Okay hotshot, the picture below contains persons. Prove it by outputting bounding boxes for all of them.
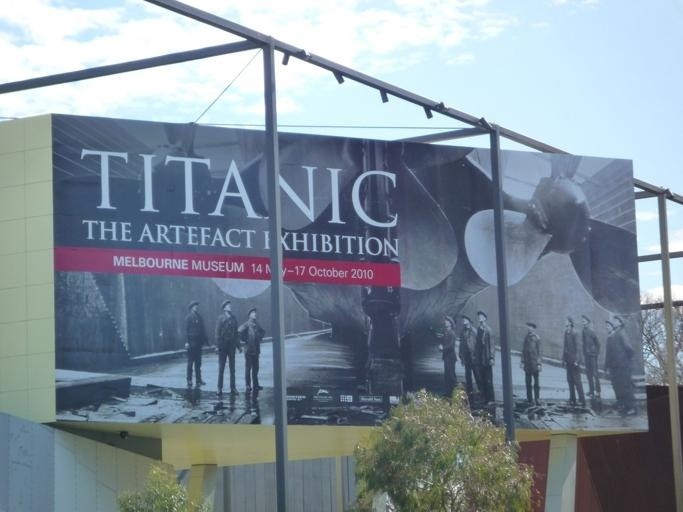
[237,307,266,391]
[183,300,210,387]
[561,316,587,407]
[519,322,542,407]
[603,320,625,412]
[459,313,483,407]
[612,314,637,415]
[474,310,496,407]
[214,299,243,396]
[581,314,602,397]
[429,314,458,397]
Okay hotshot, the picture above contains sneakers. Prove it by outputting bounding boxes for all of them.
[187,380,264,395]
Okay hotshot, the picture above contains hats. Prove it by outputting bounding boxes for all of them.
[526,320,537,327]
[566,315,575,324]
[188,300,199,309]
[220,299,230,308]
[445,311,486,327]
[605,315,626,328]
[247,307,257,317]
[582,314,591,322]
